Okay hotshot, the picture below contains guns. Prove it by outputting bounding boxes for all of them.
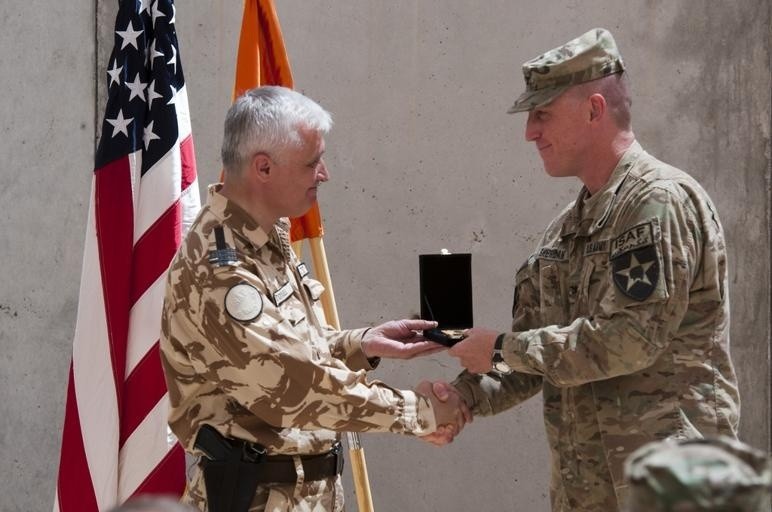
[194,423,267,512]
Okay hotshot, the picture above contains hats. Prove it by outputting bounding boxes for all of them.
[506,27,625,113]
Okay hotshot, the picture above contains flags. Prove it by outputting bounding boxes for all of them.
[47,0,200,510]
[212,2,322,274]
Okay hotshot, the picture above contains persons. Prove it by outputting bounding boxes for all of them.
[424,23,742,511]
[157,82,474,512]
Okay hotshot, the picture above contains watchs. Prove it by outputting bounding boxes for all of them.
[490,331,514,379]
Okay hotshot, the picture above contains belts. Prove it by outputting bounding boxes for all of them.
[196,442,345,482]
